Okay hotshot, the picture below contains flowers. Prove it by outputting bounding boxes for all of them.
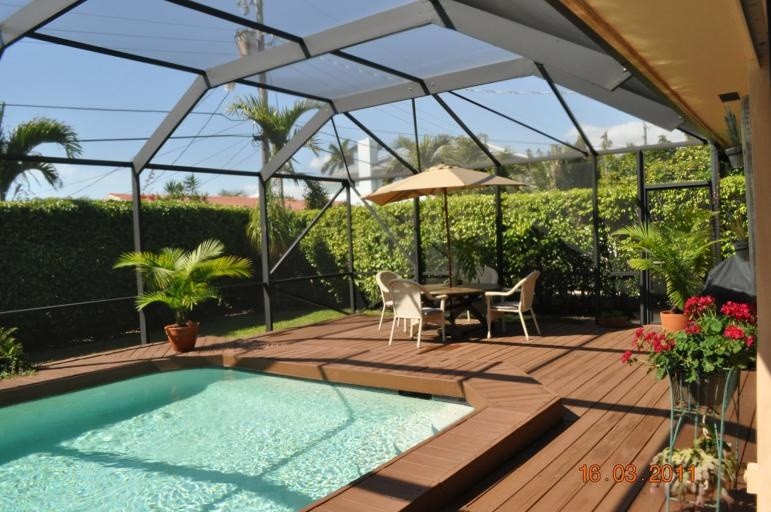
[620,294,758,388]
[650,430,743,510]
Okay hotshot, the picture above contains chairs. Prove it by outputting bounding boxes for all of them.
[375,265,542,350]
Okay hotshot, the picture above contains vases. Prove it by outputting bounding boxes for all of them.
[671,373,726,408]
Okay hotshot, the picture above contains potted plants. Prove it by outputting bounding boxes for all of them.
[112,238,256,355]
[611,202,730,333]
[598,311,628,329]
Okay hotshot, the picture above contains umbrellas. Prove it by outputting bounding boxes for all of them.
[361,159,533,337]
